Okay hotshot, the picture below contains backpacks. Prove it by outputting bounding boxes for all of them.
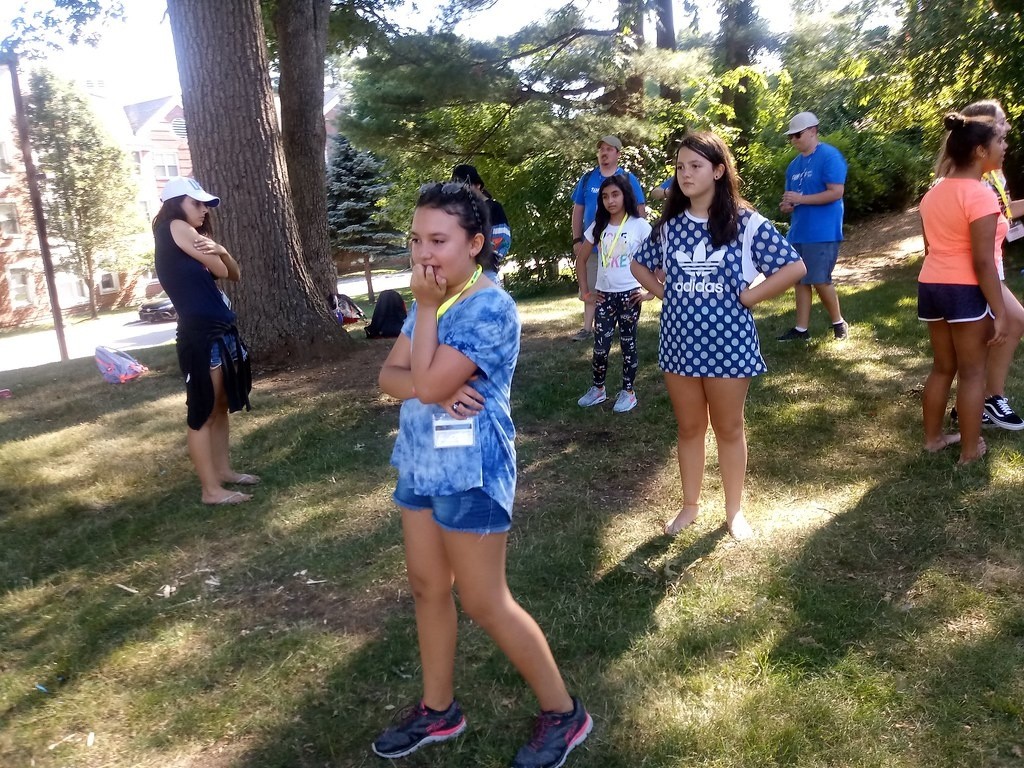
[364,290,408,339]
[94,347,146,384]
[338,292,364,322]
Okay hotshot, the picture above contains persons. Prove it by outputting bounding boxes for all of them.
[650,176,674,200]
[577,176,652,412]
[453,165,512,260]
[774,112,847,341]
[571,136,646,341]
[371,180,592,768]
[152,176,259,503]
[917,99,1024,466]
[630,132,806,543]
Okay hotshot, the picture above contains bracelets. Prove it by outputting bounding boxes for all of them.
[664,190,666,198]
[413,387,417,397]
[573,238,581,244]
[582,293,591,299]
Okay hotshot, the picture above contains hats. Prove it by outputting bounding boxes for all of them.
[783,111,820,137]
[596,136,622,153]
[452,164,485,191]
[158,176,220,207]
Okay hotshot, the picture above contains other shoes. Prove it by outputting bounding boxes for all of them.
[572,330,593,341]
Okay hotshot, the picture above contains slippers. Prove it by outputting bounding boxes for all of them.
[224,471,261,485]
[204,488,255,505]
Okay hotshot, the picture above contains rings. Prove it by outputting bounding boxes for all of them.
[452,402,460,412]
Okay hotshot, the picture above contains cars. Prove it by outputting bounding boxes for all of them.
[139,287,180,323]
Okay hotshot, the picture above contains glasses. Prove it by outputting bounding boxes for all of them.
[788,127,812,142]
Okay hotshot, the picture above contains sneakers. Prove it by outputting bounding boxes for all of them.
[372,695,467,758]
[512,694,594,768]
[983,395,1024,431]
[612,388,637,412]
[578,387,606,407]
[951,407,1002,433]
[775,327,811,343]
[834,320,848,341]
